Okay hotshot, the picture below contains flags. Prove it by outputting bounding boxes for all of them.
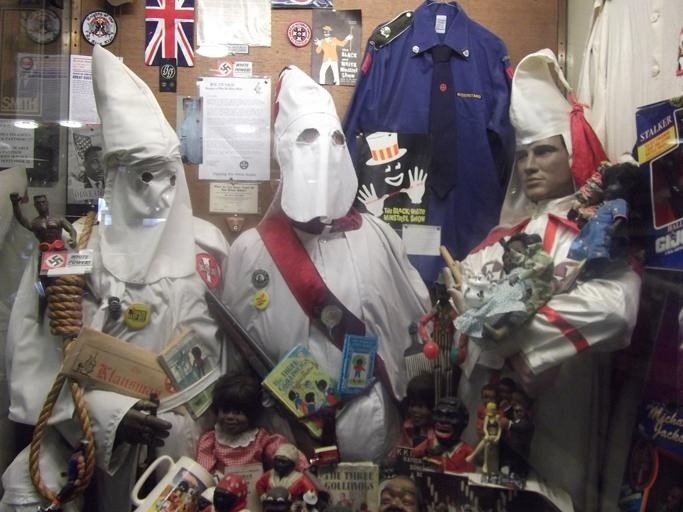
[144,0,196,68]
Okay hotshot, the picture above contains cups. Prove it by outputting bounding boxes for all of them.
[130,454,215,512]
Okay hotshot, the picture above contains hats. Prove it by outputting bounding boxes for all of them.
[506,45,584,148]
[90,41,182,163]
[272,63,341,149]
[363,130,408,168]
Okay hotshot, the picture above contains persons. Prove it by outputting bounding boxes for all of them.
[440,126,642,511]
[38,436,86,512]
[0,43,231,512]
[221,64,433,467]
[658,150,683,219]
[437,164,642,339]
[196,162,643,512]
[195,371,534,511]
[78,146,105,189]
[9,191,102,321]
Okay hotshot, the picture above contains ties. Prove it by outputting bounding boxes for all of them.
[424,45,460,205]
